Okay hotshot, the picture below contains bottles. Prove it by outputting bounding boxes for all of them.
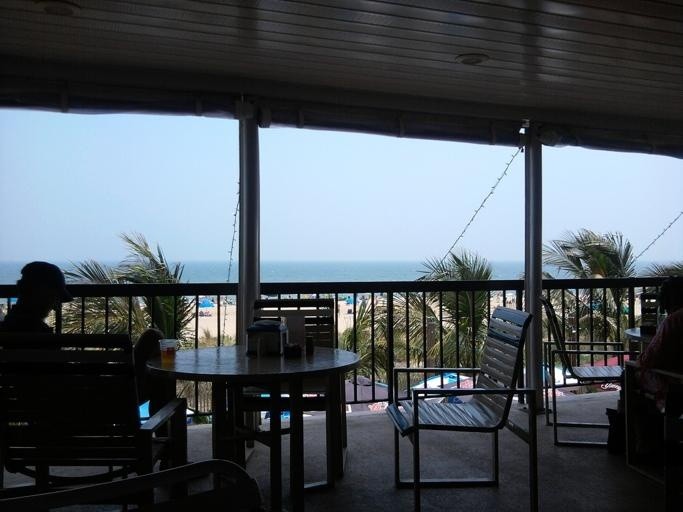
[305,336,315,356]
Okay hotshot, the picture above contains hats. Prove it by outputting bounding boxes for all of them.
[16,261,74,303]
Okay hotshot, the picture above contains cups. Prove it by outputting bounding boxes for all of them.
[159,339,176,352]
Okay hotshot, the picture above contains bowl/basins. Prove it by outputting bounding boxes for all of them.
[640,326,658,337]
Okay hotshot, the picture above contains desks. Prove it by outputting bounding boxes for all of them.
[147,341,361,505]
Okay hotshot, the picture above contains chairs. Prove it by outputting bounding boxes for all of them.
[543,299,641,448]
[624,299,680,486]
[254,298,336,463]
[394,304,538,510]
[2,322,188,471]
[2,459,264,511]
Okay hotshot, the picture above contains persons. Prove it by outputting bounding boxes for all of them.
[614,272,683,455]
[0,259,189,469]
